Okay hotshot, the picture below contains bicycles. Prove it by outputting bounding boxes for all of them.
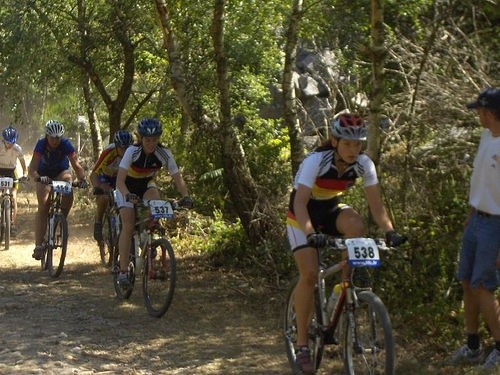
[111,190,185,317]
[91,172,150,281]
[35,175,88,278]
[0,173,30,250]
[280,225,397,374]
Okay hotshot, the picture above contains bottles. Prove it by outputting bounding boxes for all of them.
[140,232,149,250]
[326,282,349,314]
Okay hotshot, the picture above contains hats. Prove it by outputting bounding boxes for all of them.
[466,87,500,109]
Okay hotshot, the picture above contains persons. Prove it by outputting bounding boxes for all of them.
[284,108,406,374]
[1,128,28,229]
[90,130,146,243]
[114,116,193,284]
[447,85,500,374]
[28,118,90,260]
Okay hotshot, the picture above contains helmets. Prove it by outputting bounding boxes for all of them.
[329,108,369,142]
[114,129,134,147]
[136,118,163,138]
[2,127,18,143]
[44,120,66,138]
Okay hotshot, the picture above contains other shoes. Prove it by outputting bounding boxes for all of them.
[32,248,43,260]
[94,216,103,240]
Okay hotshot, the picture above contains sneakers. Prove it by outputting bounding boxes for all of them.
[481,350,500,369]
[116,271,130,285]
[447,343,485,364]
[295,341,313,374]
